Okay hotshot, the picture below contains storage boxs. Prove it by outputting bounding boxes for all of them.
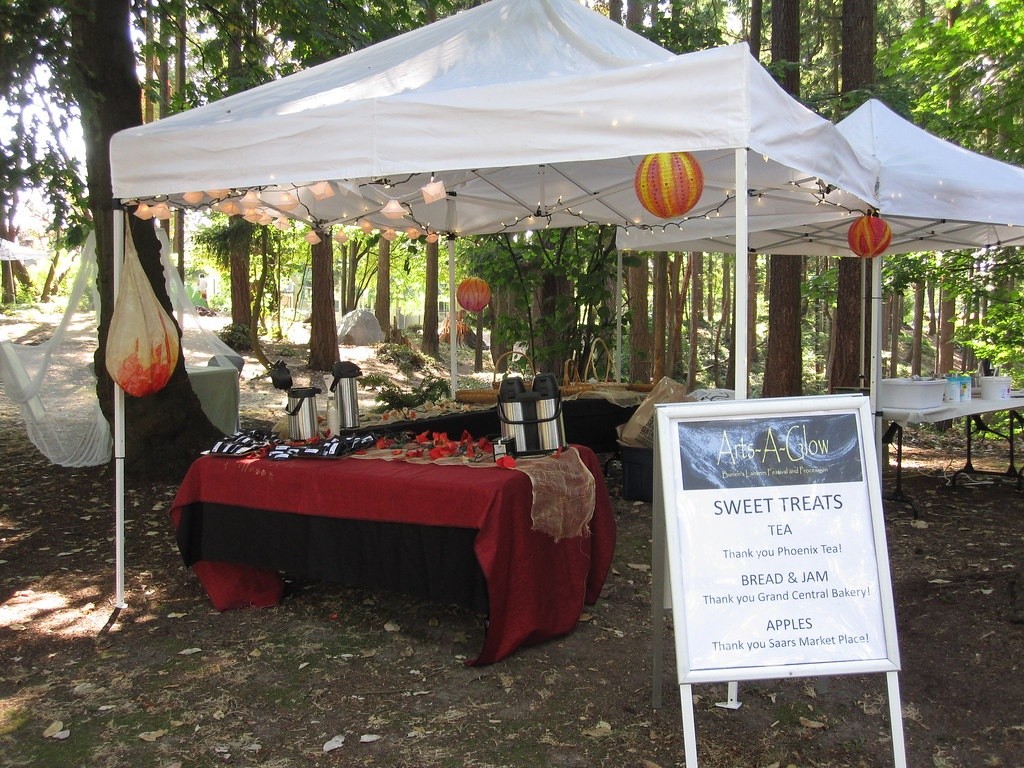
[882,377,949,409]
[620,442,653,503]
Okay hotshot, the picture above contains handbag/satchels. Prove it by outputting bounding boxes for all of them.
[104,209,180,397]
[623,375,699,447]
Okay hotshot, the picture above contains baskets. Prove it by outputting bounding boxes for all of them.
[455,338,654,404]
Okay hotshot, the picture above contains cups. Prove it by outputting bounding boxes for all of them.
[981,377,1001,401]
[961,377,972,400]
[1001,377,1010,400]
[945,377,961,401]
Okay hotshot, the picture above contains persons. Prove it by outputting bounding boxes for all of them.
[197,274,208,303]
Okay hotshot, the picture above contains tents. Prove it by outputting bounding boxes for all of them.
[110,0,882,607]
[616,97,1024,491]
[0,238,46,323]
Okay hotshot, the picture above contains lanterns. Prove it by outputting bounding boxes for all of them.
[457,276,491,312]
[848,216,892,259]
[636,152,703,219]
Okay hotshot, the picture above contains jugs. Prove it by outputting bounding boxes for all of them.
[532,374,567,450]
[271,360,322,442]
[329,361,364,427]
[497,379,530,453]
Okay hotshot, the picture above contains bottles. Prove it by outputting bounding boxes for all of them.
[327,399,339,439]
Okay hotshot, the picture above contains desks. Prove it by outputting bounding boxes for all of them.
[172,440,617,666]
[345,395,646,455]
[878,392,1024,521]
